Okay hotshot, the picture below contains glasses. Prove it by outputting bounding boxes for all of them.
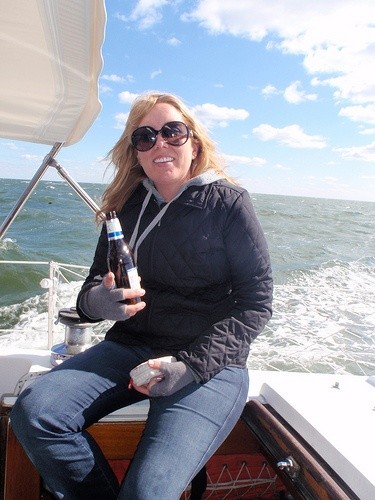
[131,121,193,152]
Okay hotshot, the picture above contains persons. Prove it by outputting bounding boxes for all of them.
[11,92,273,500]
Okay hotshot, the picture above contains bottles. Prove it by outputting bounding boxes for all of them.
[106,211,141,305]
